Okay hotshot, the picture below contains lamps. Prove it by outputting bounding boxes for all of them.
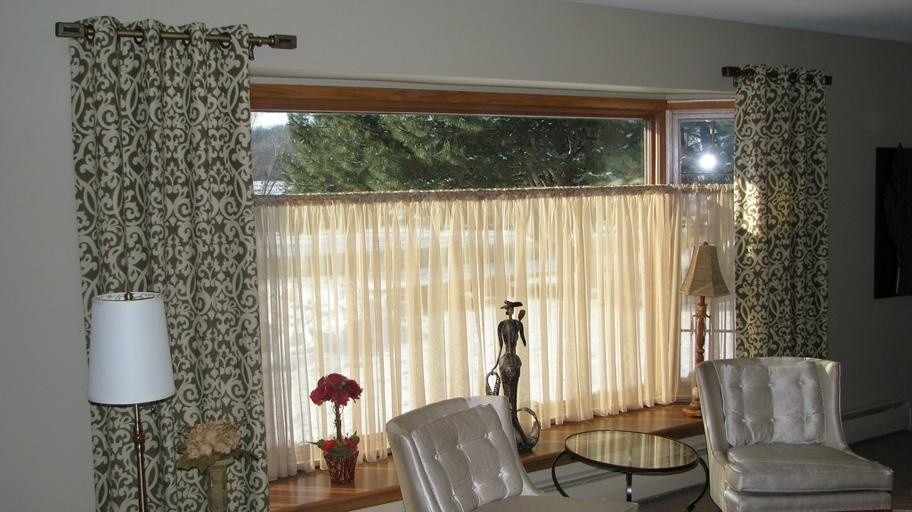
[87,291,177,511]
[678,241,731,418]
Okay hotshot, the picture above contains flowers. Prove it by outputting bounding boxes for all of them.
[307,373,363,456]
[174,421,259,476]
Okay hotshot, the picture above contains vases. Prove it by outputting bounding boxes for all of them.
[323,450,359,485]
[206,465,228,511]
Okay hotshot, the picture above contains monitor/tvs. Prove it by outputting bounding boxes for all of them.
[873,146,912,299]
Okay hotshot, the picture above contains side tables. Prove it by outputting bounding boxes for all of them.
[550,429,709,511]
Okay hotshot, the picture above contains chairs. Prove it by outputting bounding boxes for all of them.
[694,356,895,511]
[385,395,639,511]
